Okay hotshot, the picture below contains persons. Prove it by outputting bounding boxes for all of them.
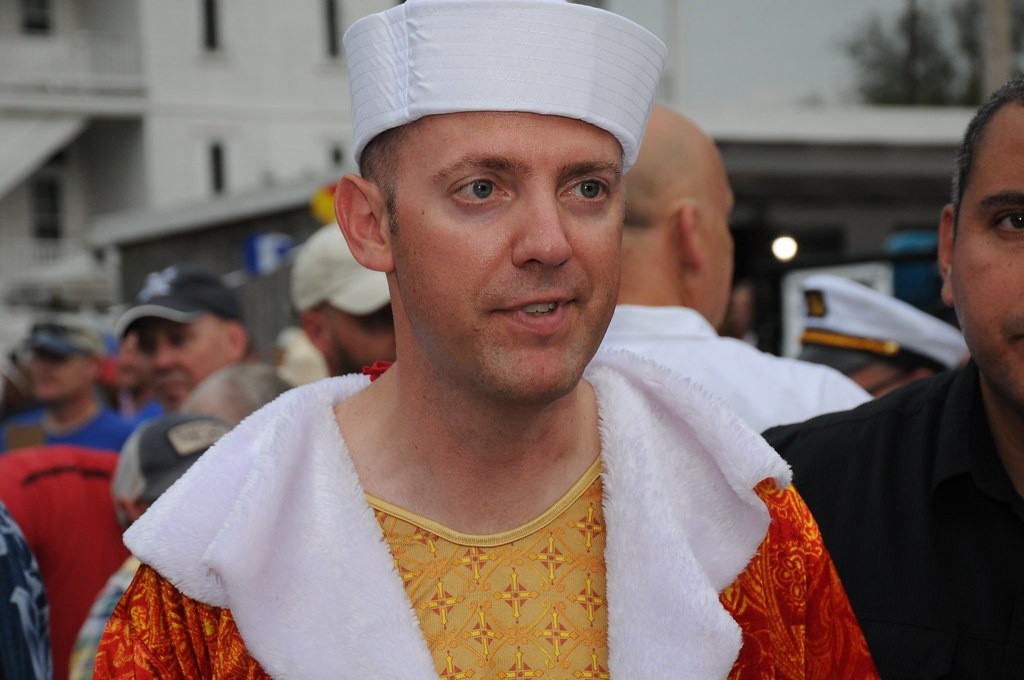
[0,108,1024,680]
[91,0,873,680]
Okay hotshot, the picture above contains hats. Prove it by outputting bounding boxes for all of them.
[113,411,236,527]
[19,316,106,358]
[289,221,395,316]
[796,276,970,373]
[114,270,245,342]
[343,0,668,175]
